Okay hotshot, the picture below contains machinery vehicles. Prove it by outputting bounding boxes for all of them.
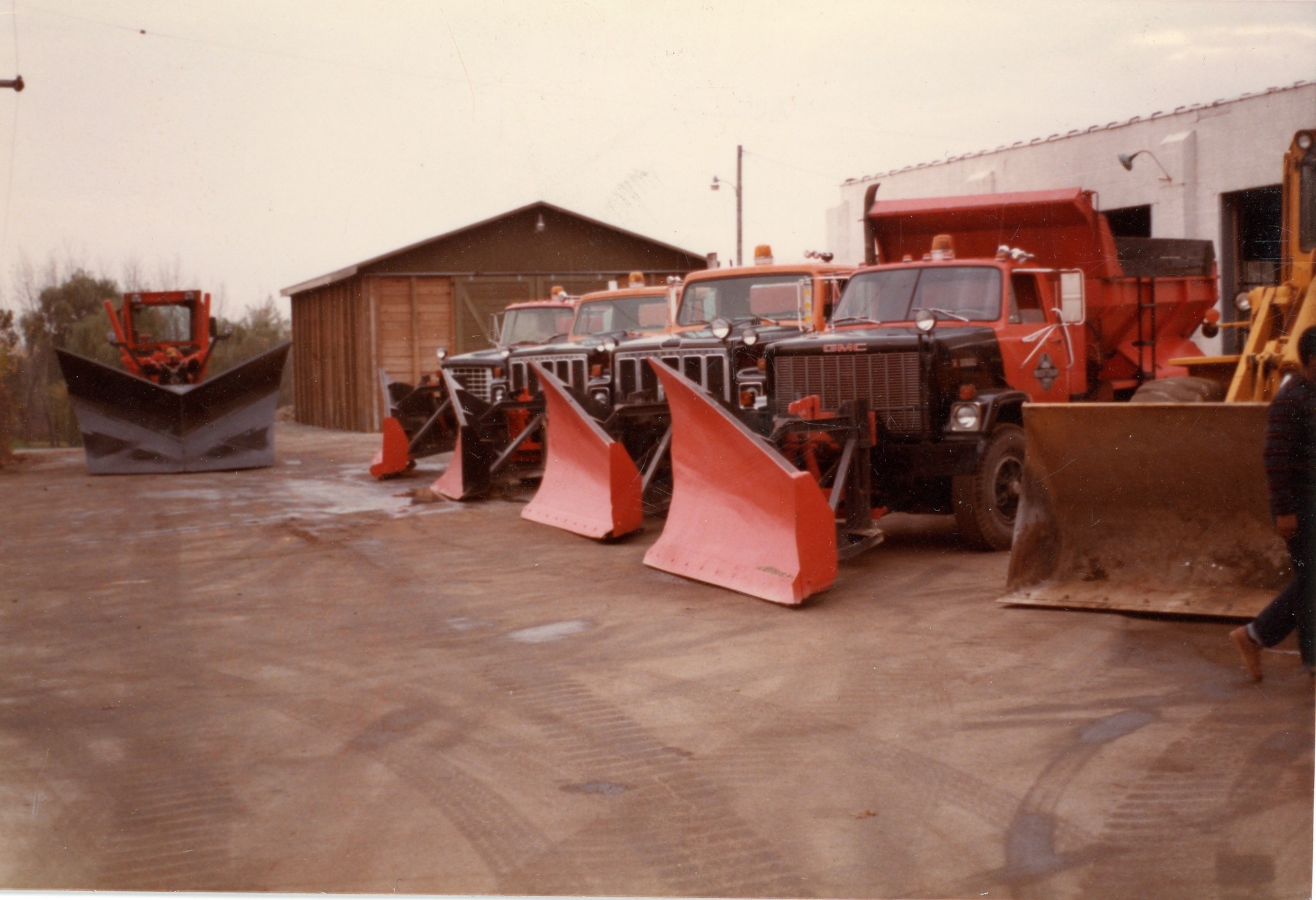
[371,125,1316,621]
[54,291,293,475]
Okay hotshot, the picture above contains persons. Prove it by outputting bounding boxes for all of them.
[1229,324,1316,685]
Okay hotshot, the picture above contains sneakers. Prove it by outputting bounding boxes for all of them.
[1229,626,1267,682]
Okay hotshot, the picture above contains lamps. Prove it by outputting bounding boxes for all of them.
[1115,147,1173,183]
